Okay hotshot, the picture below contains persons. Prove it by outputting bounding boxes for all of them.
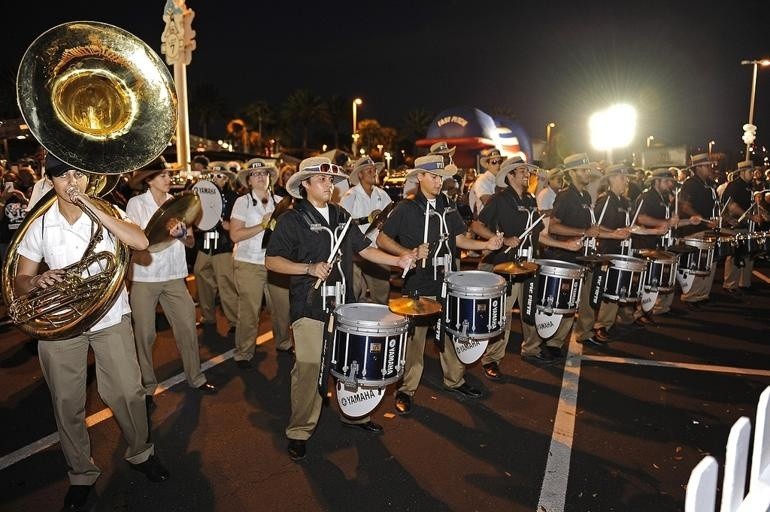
[263,143,770,410]
[265,157,419,461]
[0,140,286,412]
[15,151,179,509]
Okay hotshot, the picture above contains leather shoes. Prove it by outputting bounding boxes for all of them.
[483,362,500,380]
[228,326,235,334]
[195,321,216,328]
[131,455,170,482]
[616,315,654,329]
[547,346,562,357]
[395,392,412,415]
[200,382,216,393]
[277,344,295,354]
[687,299,709,310]
[340,421,383,434]
[287,439,306,460]
[452,383,483,398]
[576,327,609,346]
[521,353,552,363]
[725,286,743,297]
[64,485,90,510]
[238,360,250,368]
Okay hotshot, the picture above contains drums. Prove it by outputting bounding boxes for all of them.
[330,304,411,420]
[533,259,586,339]
[641,252,677,313]
[677,237,715,294]
[601,254,646,305]
[764,232,770,253]
[186,174,228,231]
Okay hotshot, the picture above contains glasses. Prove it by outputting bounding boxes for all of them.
[302,163,340,174]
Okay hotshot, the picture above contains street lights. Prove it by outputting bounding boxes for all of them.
[708,140,715,157]
[646,135,654,148]
[319,95,409,176]
[547,122,555,143]
[740,57,770,160]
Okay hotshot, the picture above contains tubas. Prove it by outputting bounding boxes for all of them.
[0,20,179,342]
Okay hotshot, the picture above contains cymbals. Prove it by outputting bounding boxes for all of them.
[494,255,537,275]
[388,290,443,317]
[144,189,201,252]
[363,200,399,237]
[261,195,292,249]
[671,243,692,253]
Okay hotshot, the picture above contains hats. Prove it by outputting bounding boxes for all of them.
[285,156,349,200]
[45,151,77,176]
[405,141,457,184]
[130,155,184,190]
[201,158,280,188]
[349,156,385,185]
[734,160,756,173]
[479,149,538,188]
[642,152,715,185]
[547,152,638,191]
